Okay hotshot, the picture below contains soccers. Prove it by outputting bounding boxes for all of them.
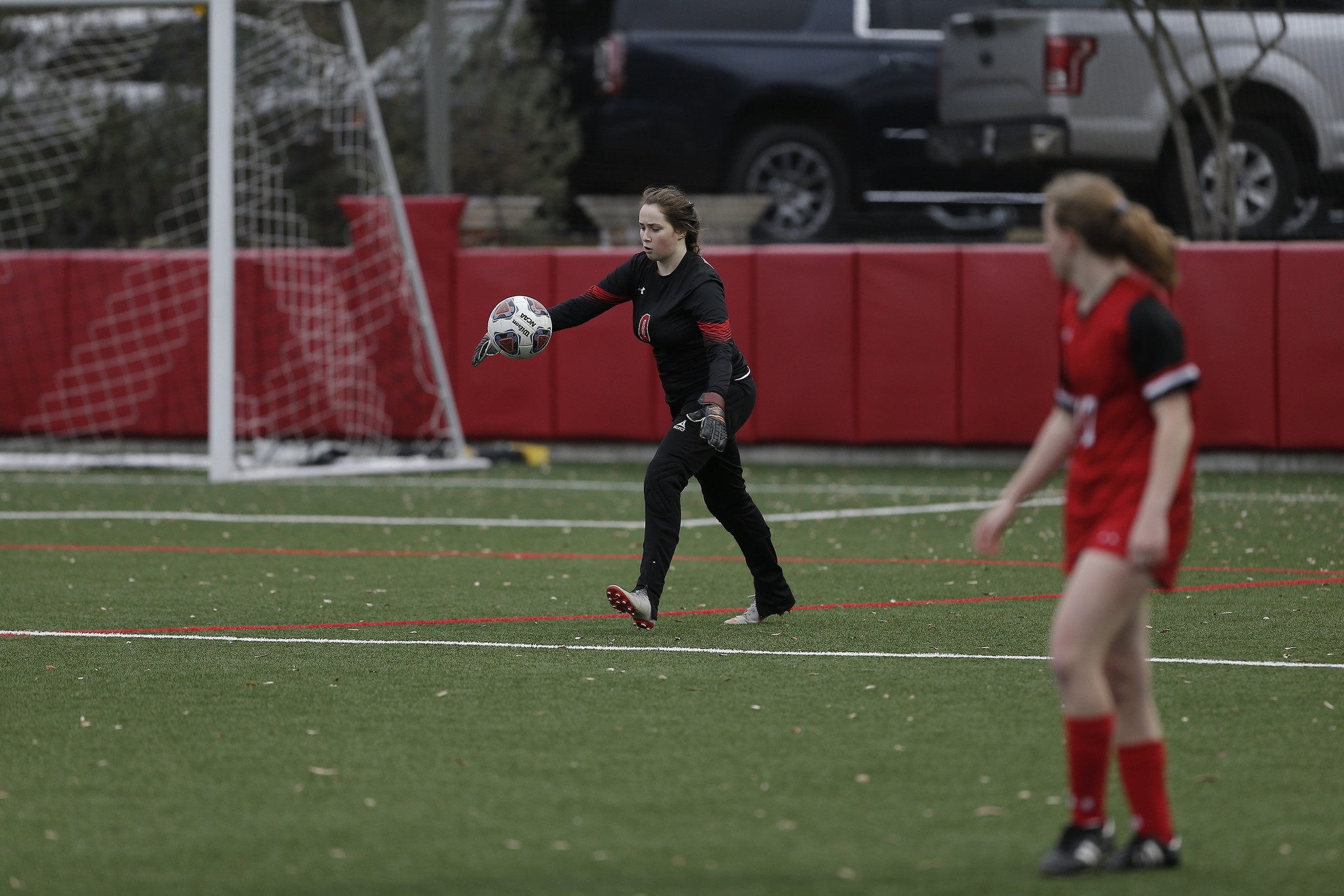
[487,294,552,359]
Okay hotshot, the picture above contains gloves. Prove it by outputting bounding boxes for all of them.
[472,331,499,368]
[687,391,728,452]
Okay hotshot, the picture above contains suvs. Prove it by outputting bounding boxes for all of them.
[10,0,1344,237]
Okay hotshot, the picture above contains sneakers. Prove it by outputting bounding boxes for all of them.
[1038,822,1112,876]
[607,584,656,631]
[1106,833,1181,871]
[723,593,795,625]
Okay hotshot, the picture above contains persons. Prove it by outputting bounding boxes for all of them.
[472,186,796,629]
[972,171,1208,880]
